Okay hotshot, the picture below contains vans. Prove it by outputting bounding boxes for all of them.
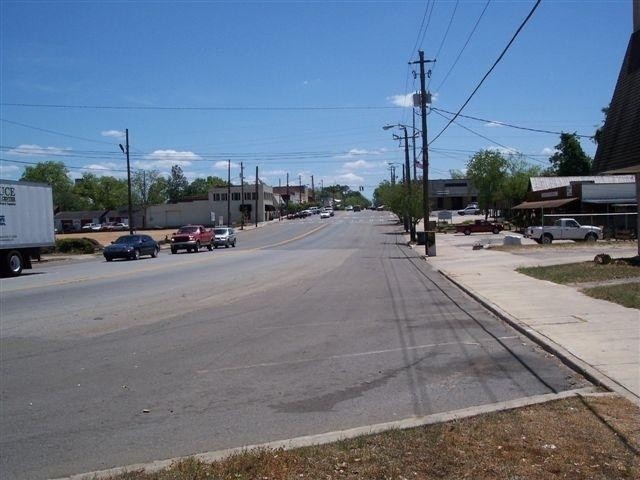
[213,227,237,248]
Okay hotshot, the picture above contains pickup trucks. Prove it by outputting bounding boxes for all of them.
[170,224,216,254]
[524,218,604,244]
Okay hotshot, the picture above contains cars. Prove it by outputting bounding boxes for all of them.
[345,204,393,212]
[287,206,335,220]
[103,234,161,261]
[457,205,481,216]
[81,221,129,232]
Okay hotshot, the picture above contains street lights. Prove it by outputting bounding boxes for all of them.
[387,162,405,182]
[383,124,430,230]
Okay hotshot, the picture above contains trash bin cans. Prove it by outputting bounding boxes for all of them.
[417,232,424,245]
[429,221,436,230]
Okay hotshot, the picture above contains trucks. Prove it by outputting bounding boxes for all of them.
[455,218,504,235]
[0,179,56,277]
